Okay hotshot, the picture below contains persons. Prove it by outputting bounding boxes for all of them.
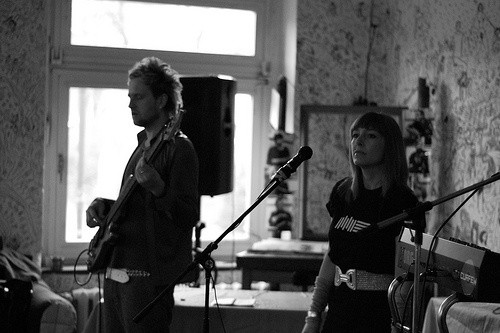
[85,57,198,333]
[301,112,414,333]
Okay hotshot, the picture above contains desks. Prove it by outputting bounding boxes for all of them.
[82,290,328,333]
[236,249,324,290]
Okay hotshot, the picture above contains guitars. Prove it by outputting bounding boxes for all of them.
[87,107,184,275]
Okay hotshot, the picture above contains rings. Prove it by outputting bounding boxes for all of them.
[141,170,144,173]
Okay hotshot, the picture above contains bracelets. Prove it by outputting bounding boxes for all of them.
[307,310,320,318]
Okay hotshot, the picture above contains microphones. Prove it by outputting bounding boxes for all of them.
[267,145,313,183]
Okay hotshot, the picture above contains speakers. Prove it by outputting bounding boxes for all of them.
[178,76,234,195]
